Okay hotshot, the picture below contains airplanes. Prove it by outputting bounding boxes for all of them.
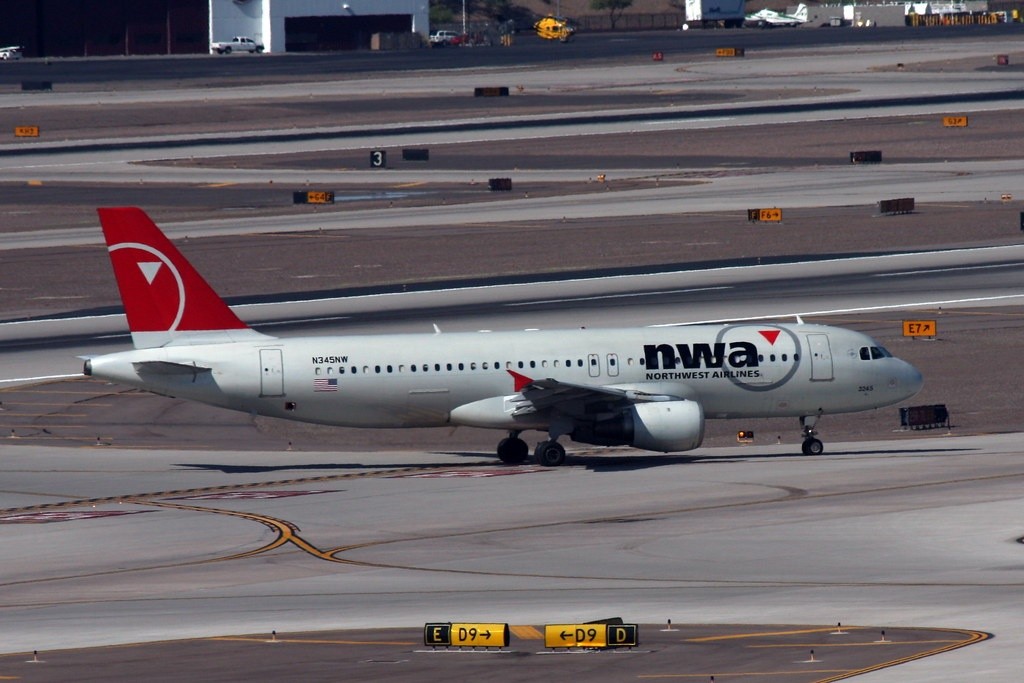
[75,206,923,467]
[744,2,813,29]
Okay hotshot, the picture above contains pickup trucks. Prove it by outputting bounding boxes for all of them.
[211,36,265,53]
[0,50,23,60]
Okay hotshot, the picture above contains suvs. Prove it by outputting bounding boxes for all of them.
[430,29,460,47]
